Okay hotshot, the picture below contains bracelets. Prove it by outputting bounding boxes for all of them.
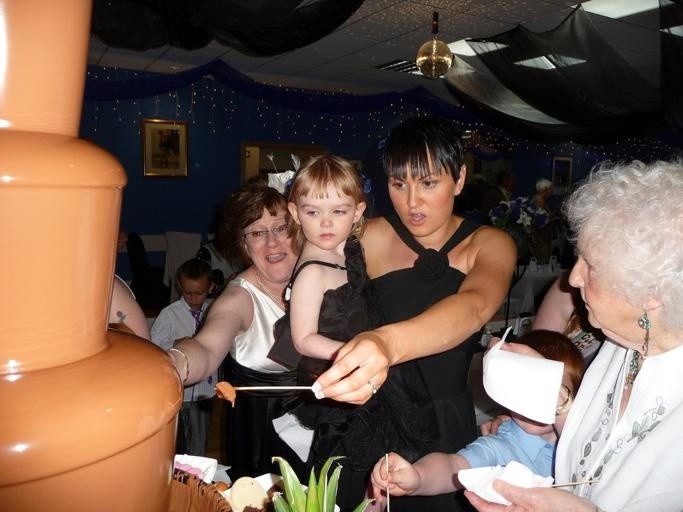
[167,347,191,386]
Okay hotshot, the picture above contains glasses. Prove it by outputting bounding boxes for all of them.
[243,224,290,241]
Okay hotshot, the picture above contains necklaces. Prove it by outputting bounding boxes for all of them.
[623,351,645,389]
[255,274,292,311]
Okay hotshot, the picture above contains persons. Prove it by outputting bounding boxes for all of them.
[370,329,586,512]
[463,158,681,512]
[159,175,299,481]
[311,112,518,512]
[151,258,221,457]
[268,154,443,510]
[110,277,151,344]
[530,269,603,370]
[455,163,577,266]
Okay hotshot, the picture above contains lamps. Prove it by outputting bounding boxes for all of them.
[415,12,453,77]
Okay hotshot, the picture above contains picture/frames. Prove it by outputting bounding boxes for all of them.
[550,156,574,196]
[240,140,327,187]
[349,160,362,172]
[141,119,190,181]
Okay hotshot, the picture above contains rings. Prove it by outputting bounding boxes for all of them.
[367,382,380,398]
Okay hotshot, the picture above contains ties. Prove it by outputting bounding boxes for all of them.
[190,309,212,385]
